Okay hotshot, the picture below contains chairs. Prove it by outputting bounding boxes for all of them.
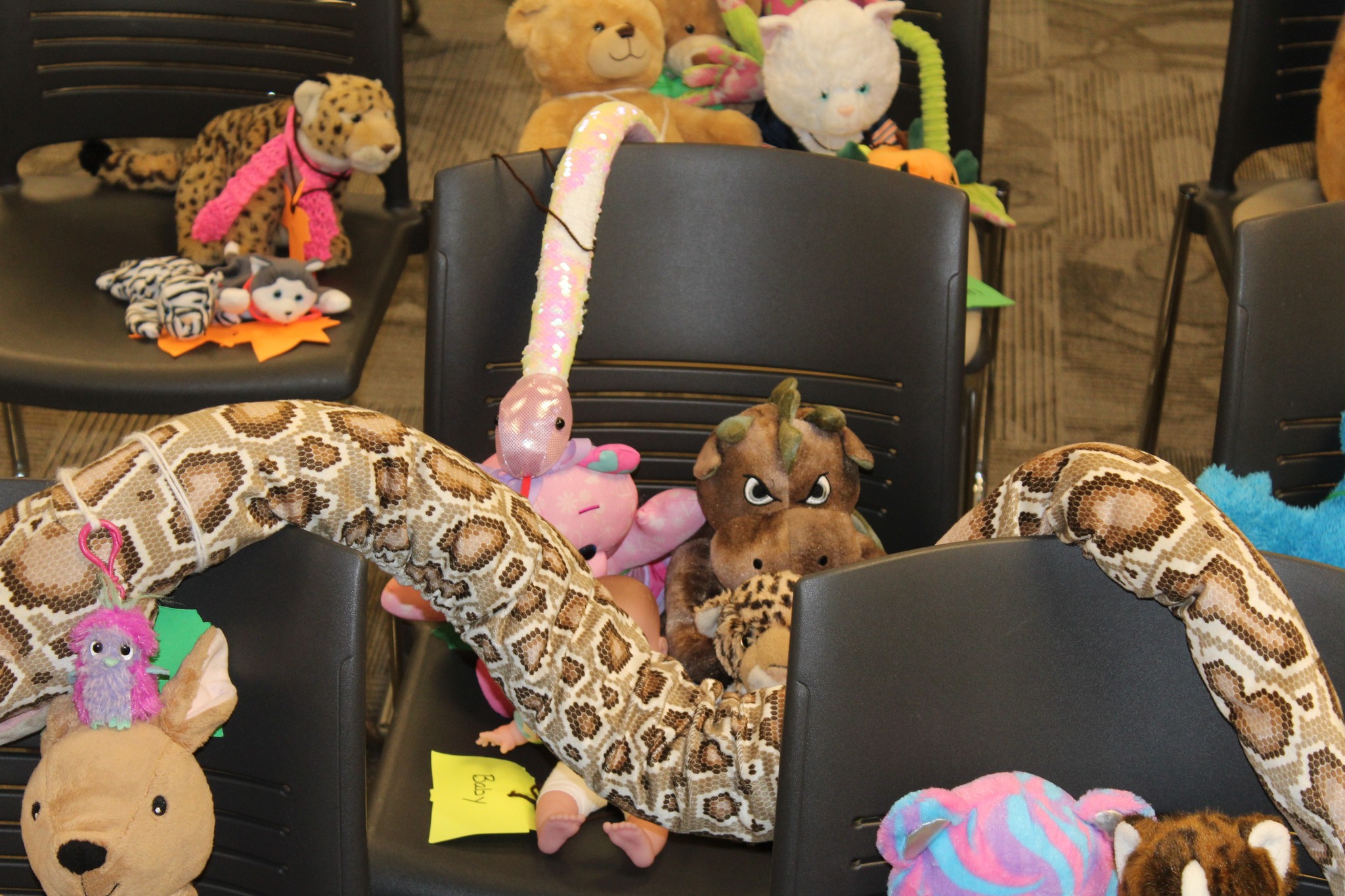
[1211,198,1345,512]
[369,137,973,896]
[1132,0,1345,454]
[760,534,1345,896]
[1,0,433,477]
[0,478,367,895]
[755,0,1014,504]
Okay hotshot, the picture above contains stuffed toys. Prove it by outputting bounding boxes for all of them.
[0,0,1345,896]
